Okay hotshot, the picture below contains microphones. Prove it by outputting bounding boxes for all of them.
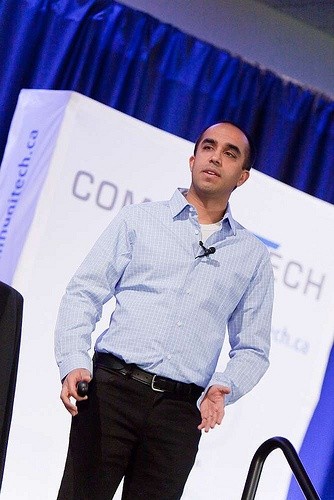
[196,241,216,259]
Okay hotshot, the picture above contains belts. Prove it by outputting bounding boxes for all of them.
[92,352,203,404]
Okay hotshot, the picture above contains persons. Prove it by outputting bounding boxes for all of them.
[53,121,275,500]
